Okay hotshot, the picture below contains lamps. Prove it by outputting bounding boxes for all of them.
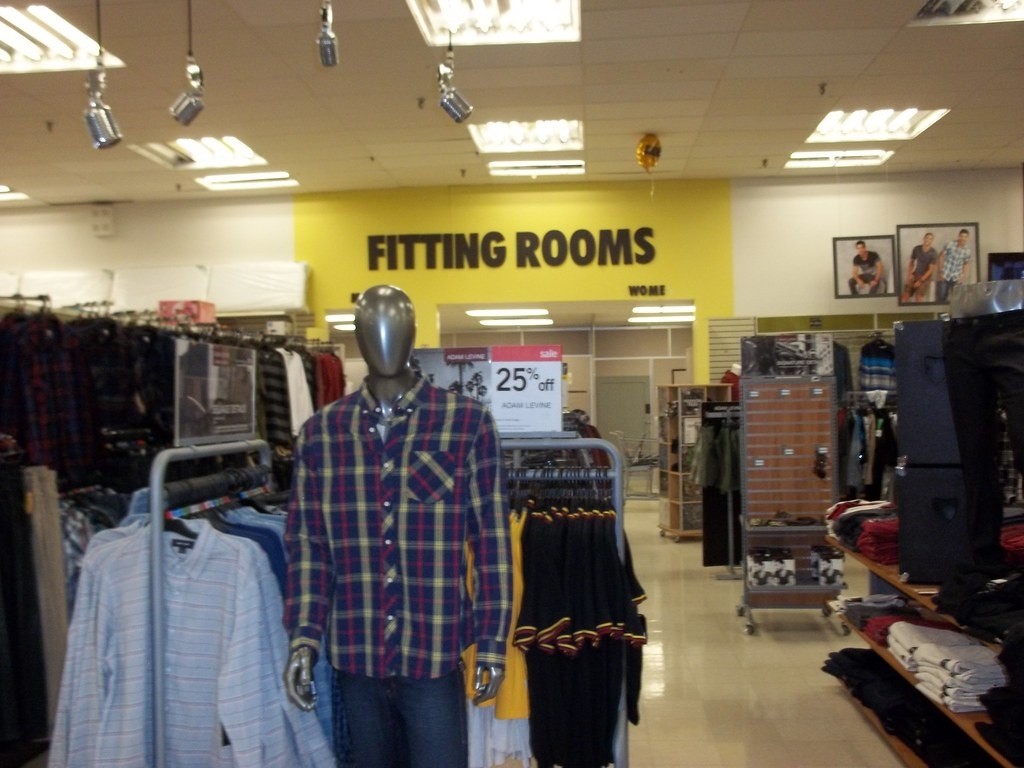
[81,0,474,150]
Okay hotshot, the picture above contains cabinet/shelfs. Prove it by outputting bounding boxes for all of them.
[823,536,1024,768]
[743,519,846,594]
[654,382,735,543]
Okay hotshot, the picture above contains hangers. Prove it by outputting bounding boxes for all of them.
[509,407,615,512]
[7,293,339,541]
[699,331,897,433]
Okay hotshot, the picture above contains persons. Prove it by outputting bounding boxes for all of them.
[901,229,938,304]
[938,229,972,304]
[849,242,883,295]
[282,285,514,768]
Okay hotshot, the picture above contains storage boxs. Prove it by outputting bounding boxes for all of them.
[266,321,292,336]
[159,301,216,325]
[894,320,961,466]
[899,466,1003,585]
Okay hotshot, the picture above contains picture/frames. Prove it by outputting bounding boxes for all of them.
[832,234,897,299]
[987,253,1024,281]
[896,222,983,306]
